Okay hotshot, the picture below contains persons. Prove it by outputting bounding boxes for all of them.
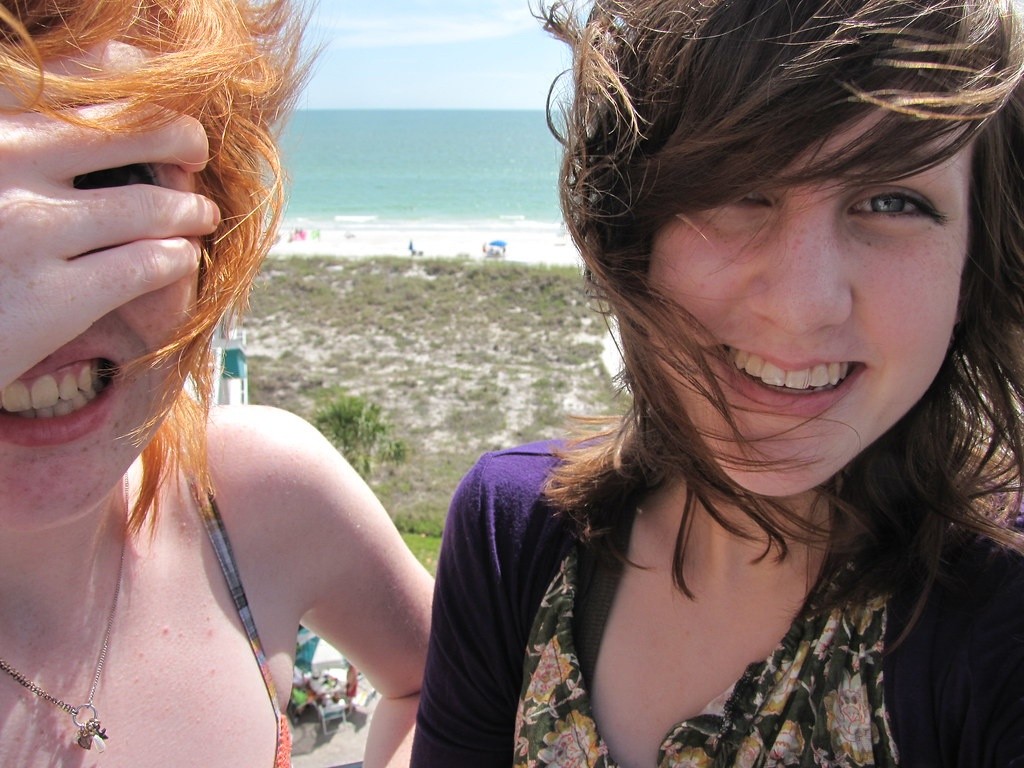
[410,0,1024,768]
[0,1,436,767]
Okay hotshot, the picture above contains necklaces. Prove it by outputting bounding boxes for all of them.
[0,470,128,753]
